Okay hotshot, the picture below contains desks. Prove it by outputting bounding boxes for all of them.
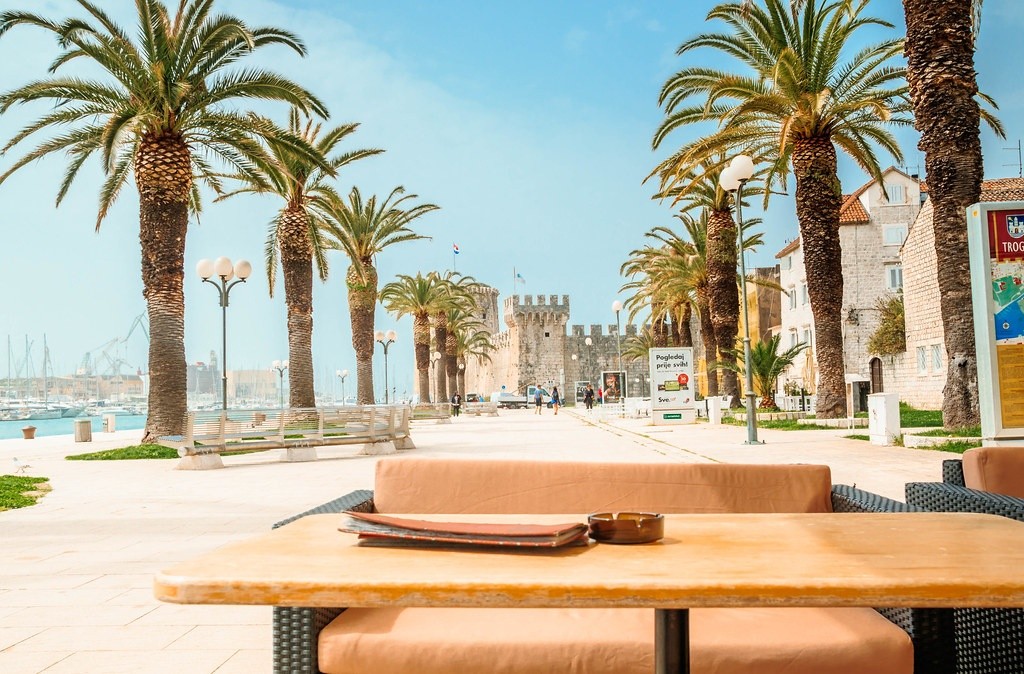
[154,512,1024,674]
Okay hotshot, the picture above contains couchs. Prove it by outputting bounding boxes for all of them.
[904,447,1024,674]
[272,458,957,674]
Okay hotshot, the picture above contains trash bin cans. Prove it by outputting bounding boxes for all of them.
[75,419,91,442]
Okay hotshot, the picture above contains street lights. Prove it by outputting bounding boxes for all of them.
[572,354,578,385]
[375,330,397,403]
[430,352,442,404]
[336,370,347,404]
[719,154,758,448]
[198,257,254,449]
[612,300,624,404]
[635,373,650,397]
[272,360,289,411]
[585,338,593,385]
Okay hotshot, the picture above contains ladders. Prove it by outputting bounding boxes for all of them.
[503,380,531,398]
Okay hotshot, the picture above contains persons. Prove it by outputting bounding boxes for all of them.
[451,391,461,417]
[534,385,544,415]
[551,386,559,415]
[584,383,594,411]
[599,386,602,404]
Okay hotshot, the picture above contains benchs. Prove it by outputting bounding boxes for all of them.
[408,403,454,426]
[463,402,499,417]
[157,406,416,469]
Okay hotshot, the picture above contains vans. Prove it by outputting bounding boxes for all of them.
[490,393,512,408]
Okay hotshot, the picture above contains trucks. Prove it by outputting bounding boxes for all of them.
[496,386,553,409]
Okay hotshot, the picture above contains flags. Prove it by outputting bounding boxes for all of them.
[453,243,459,254]
[517,274,525,283]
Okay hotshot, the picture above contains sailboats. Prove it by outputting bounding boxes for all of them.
[0,332,147,421]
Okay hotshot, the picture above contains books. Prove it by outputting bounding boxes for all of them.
[337,510,587,547]
